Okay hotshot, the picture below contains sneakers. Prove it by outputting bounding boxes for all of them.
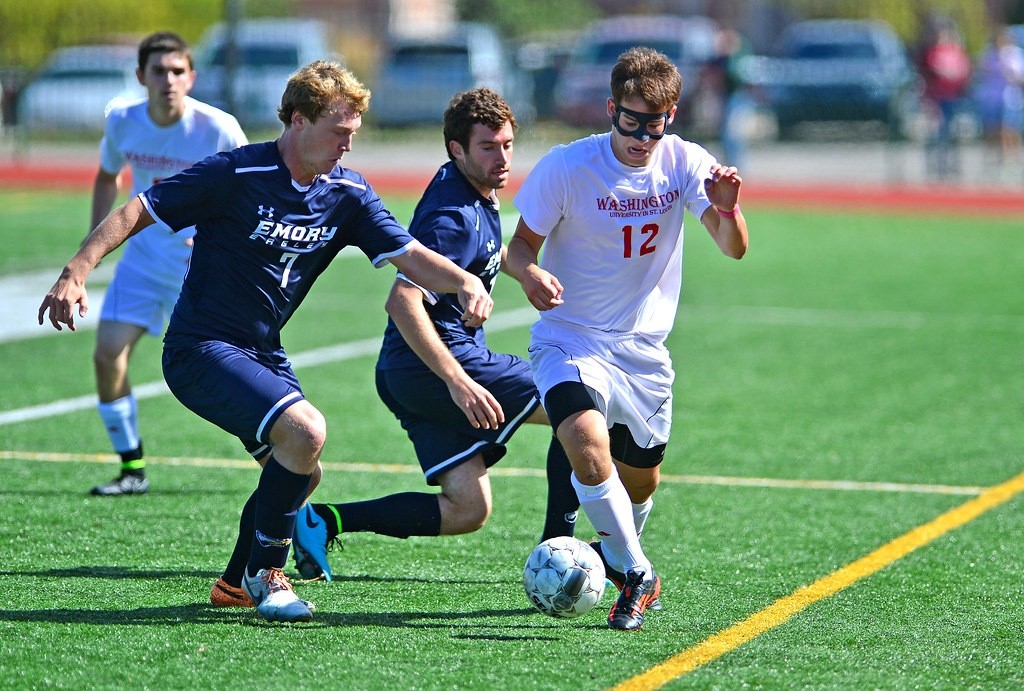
[589,541,662,610]
[607,564,660,631]
[293,502,344,582]
[241,566,312,622]
[210,574,315,610]
[91,473,150,496]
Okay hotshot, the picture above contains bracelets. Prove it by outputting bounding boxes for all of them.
[715,203,741,218]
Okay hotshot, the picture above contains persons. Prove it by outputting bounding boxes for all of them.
[35,60,496,624]
[917,12,1024,186]
[292,85,582,584]
[704,26,760,172]
[79,31,251,498]
[507,49,750,631]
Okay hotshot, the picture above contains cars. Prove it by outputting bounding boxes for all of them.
[185,17,348,129]
[365,21,533,122]
[15,44,147,139]
[941,20,1023,143]
[741,18,919,143]
[550,14,726,136]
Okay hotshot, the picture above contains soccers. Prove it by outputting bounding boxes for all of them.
[523,536,606,619]
